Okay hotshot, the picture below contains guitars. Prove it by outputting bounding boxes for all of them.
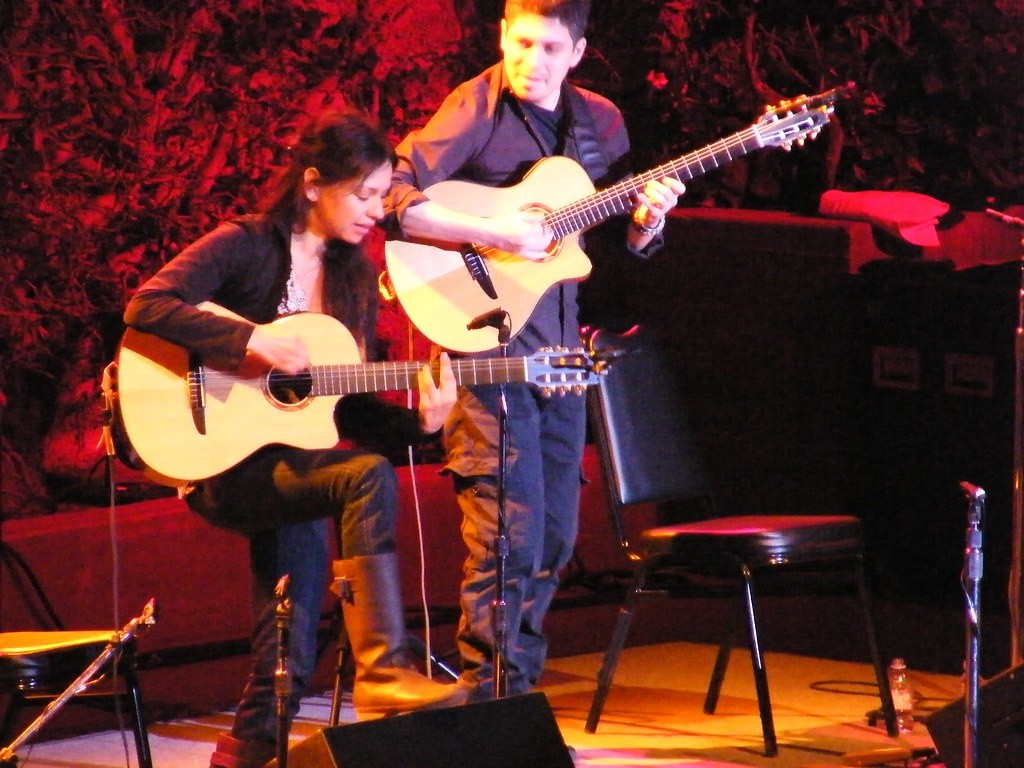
[358,80,860,357]
[102,300,612,488]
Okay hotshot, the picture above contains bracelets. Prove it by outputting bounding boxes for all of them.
[632,210,665,236]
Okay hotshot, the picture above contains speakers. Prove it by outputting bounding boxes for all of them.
[262,691,578,768]
[925,661,1023,768]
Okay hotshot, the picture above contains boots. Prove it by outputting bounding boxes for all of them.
[210,731,278,768]
[329,552,469,722]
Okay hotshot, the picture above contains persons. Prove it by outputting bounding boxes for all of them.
[377,0,685,707]
[123,115,470,768]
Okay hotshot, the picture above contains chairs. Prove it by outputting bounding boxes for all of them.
[583,357,901,755]
[1,541,155,766]
